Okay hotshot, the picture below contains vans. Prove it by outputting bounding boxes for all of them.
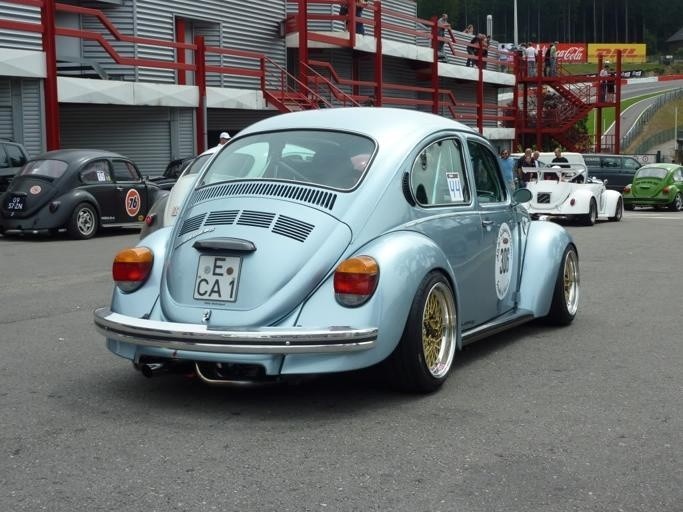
[581,154,643,193]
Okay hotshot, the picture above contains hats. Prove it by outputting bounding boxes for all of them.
[220,132,231,139]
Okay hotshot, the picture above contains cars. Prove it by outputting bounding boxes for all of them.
[0,142,170,240]
[91,105,580,397]
[621,162,683,212]
[139,140,316,240]
[0,136,33,193]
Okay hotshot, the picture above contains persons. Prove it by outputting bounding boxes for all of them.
[599,65,611,102]
[607,74,616,103]
[436,14,451,53]
[216,132,231,146]
[466,33,559,78]
[499,147,570,190]
[462,25,473,35]
[338,0,374,35]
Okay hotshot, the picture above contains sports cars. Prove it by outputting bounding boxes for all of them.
[512,159,624,226]
